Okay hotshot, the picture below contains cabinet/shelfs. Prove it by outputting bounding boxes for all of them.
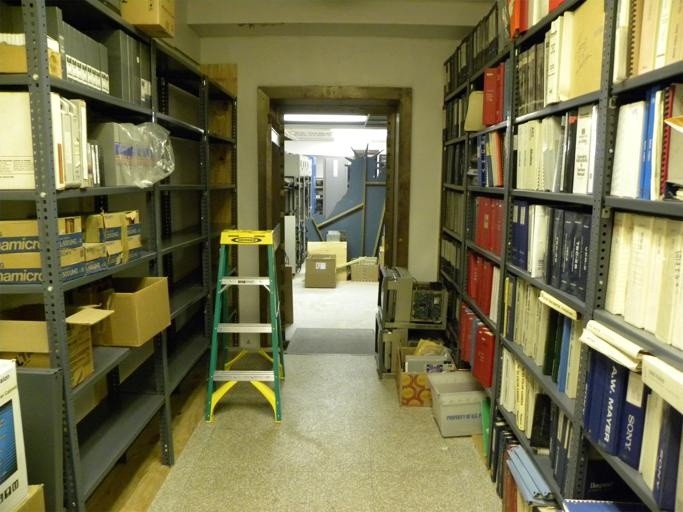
[284,175,325,277]
[442,0,683,512]
[0,0,238,512]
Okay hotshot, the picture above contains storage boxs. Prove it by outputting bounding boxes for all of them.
[284,264,294,324]
[397,346,489,438]
[305,240,380,288]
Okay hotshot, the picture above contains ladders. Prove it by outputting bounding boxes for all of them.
[205,229,285,423]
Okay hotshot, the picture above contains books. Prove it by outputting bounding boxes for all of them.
[405,18,496,386]
[0,0,163,192]
[489,1,683,512]
